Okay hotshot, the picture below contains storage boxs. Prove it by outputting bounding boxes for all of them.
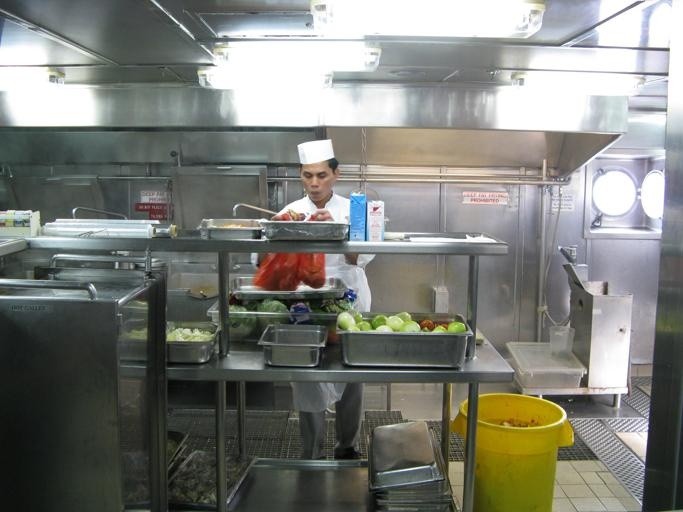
[506,341,588,389]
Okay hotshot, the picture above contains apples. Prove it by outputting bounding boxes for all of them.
[336,309,466,333]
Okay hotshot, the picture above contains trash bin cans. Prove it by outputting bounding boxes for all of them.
[449,393,575,512]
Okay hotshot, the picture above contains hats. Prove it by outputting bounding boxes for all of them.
[295,138,338,166]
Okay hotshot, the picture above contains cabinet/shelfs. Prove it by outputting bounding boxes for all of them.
[26,230,515,511]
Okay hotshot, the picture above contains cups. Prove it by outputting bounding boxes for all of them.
[549,325,576,361]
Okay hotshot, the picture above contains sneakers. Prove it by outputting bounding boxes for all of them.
[333,446,362,460]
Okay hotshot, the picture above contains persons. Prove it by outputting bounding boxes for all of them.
[250,138,376,461]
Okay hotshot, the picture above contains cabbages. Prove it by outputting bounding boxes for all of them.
[219,295,350,330]
